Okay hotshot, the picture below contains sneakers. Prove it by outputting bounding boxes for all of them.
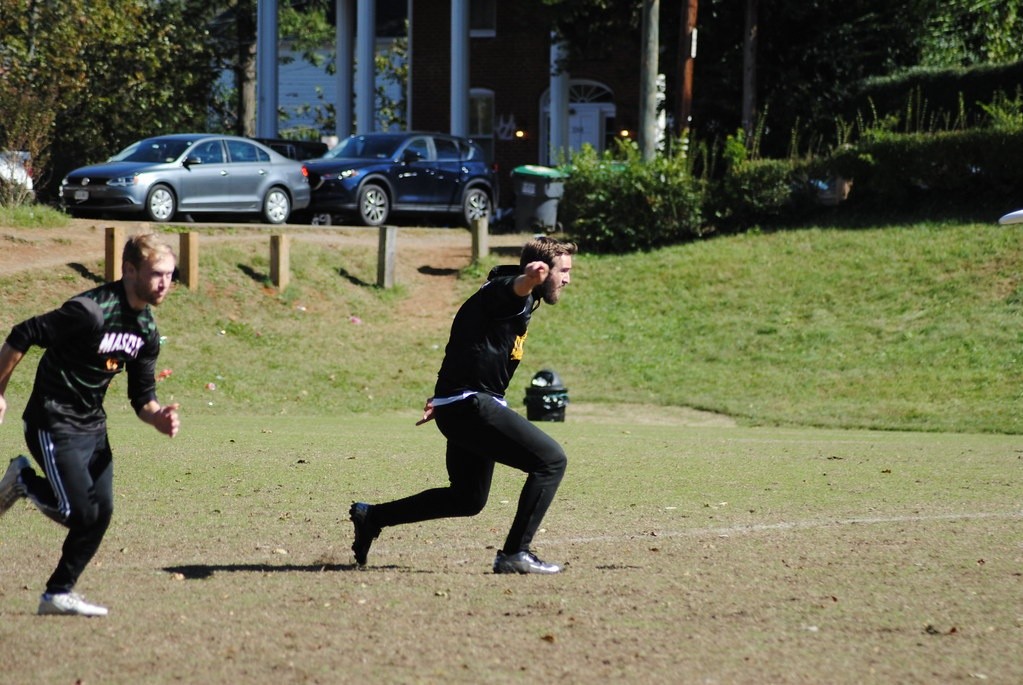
[0,455,29,515]
[494,545,562,575]
[349,502,379,565]
[38,589,109,620]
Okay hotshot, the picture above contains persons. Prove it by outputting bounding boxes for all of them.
[0,232,180,616]
[349,236,579,574]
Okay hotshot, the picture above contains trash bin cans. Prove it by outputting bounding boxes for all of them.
[509,163,571,231]
[523,369,569,422]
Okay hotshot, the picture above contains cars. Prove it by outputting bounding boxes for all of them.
[0,146,34,205]
[303,132,499,226]
[58,133,312,222]
